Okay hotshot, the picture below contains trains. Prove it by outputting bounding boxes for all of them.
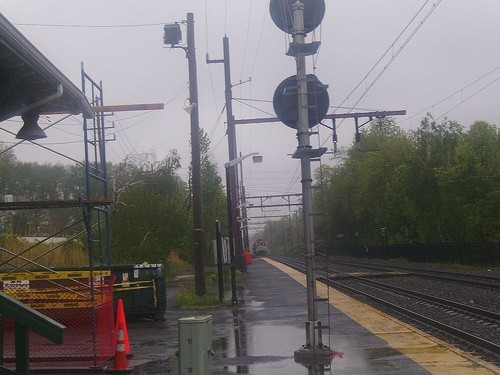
[252,238,268,256]
[0,262,167,322]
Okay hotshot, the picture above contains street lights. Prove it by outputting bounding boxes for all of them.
[225,151,262,303]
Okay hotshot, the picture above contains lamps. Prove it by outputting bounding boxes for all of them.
[164,23,190,57]
